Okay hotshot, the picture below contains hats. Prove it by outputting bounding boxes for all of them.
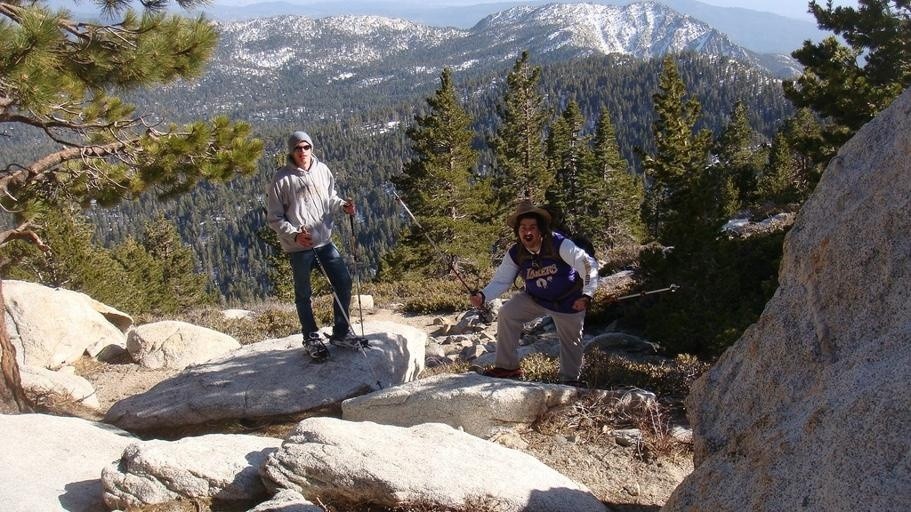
[287,131,313,152]
[507,201,551,229]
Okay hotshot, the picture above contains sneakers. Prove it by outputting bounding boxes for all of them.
[330,330,368,350]
[485,367,524,378]
[303,333,330,359]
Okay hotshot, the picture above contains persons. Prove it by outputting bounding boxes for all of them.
[465,199,601,388]
[265,130,369,360]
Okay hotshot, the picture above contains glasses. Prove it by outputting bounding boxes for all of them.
[294,145,310,150]
